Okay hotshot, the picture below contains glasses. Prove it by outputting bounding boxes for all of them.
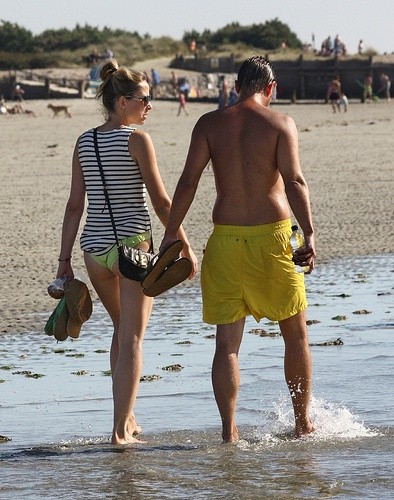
[120,94,151,106]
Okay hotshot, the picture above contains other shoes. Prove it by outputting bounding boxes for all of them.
[44,296,69,341]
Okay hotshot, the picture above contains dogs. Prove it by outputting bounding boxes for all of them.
[47,103,73,119]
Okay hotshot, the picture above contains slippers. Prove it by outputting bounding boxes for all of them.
[141,240,183,288]
[144,257,192,297]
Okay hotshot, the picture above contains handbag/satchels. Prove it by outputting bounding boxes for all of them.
[118,247,159,282]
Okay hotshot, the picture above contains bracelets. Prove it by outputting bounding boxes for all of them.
[57,256,72,262]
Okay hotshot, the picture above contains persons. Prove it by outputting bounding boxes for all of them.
[329,75,341,112]
[197,73,215,97]
[144,67,159,97]
[339,92,348,112]
[12,85,24,102]
[363,73,373,103]
[359,40,363,55]
[0,99,22,114]
[88,48,113,78]
[217,75,238,108]
[170,71,176,90]
[56,61,199,444]
[159,56,316,443]
[381,74,391,100]
[174,78,189,116]
[320,34,346,57]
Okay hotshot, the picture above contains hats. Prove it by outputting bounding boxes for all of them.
[64,279,93,338]
